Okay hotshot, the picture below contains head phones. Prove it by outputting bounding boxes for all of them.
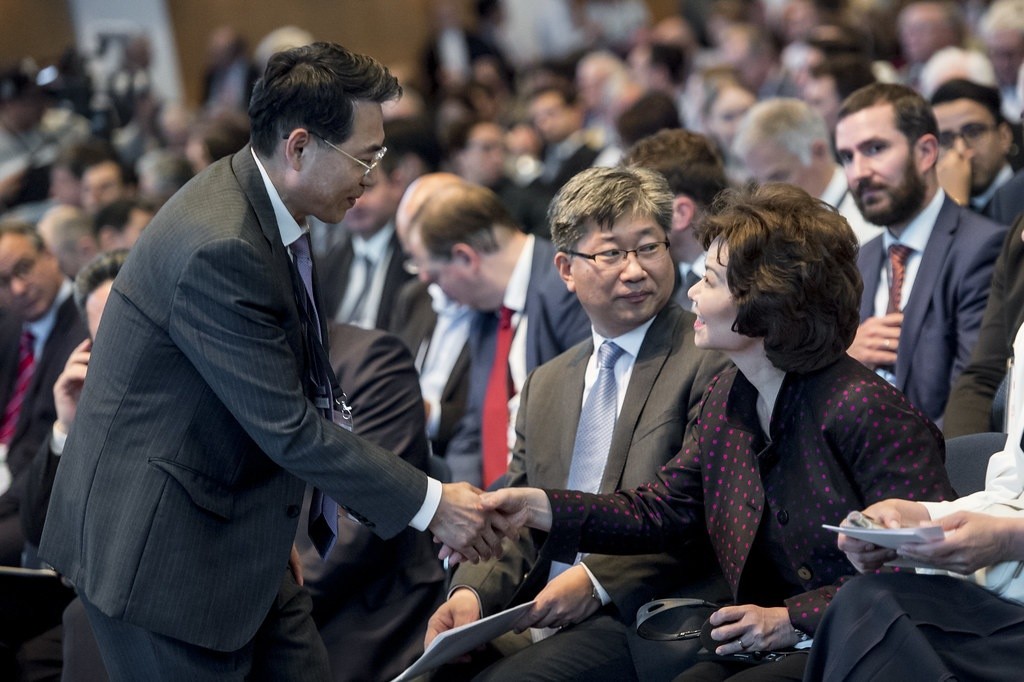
[635,597,743,652]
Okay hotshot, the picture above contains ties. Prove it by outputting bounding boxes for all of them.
[290,235,323,347]
[884,244,911,316]
[0,328,36,443]
[481,307,515,490]
[551,339,629,582]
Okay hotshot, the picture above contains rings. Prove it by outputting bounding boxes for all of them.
[885,338,889,350]
[738,639,746,649]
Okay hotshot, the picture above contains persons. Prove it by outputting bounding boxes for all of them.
[0,0,1024,682]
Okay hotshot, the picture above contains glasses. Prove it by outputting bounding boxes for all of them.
[0,243,43,289]
[937,122,1001,150]
[282,128,388,178]
[565,230,671,271]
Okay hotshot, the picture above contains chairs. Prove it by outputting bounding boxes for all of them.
[943,433,1008,496]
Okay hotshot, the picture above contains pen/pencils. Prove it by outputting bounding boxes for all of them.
[848,511,884,530]
